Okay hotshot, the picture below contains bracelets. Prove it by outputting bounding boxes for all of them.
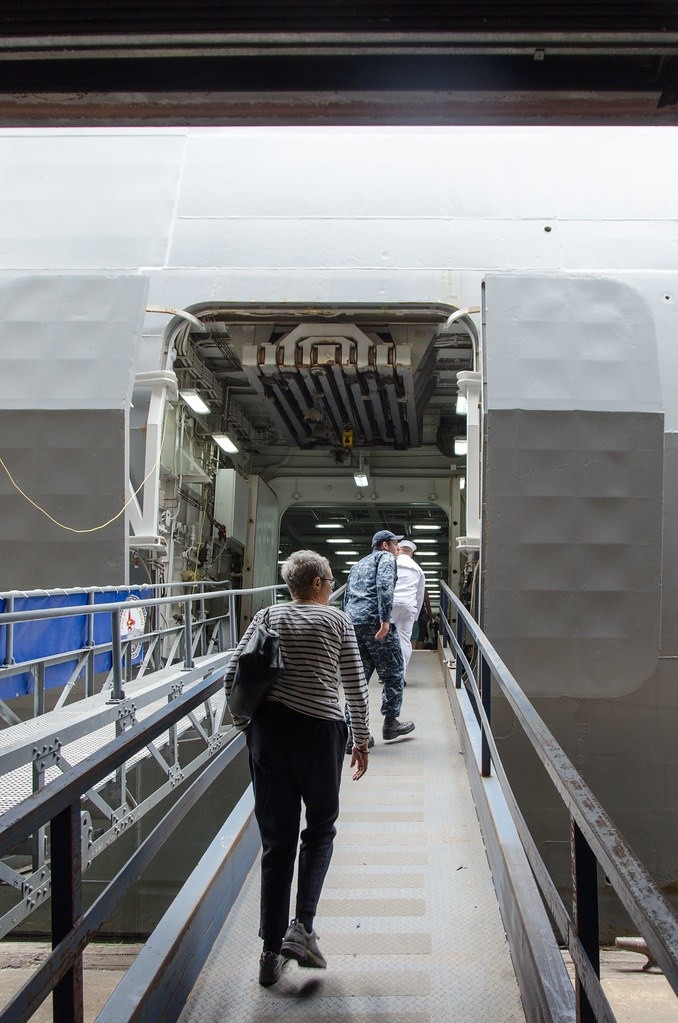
[353,745,371,755]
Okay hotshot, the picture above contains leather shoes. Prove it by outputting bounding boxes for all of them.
[383,720,415,740]
[345,736,374,753]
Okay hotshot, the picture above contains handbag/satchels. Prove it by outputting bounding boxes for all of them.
[228,607,284,721]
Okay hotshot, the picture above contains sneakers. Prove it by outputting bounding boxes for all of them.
[280,919,327,968]
[259,940,288,986]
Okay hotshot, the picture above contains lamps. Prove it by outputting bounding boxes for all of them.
[452,394,466,490]
[179,389,211,415]
[353,455,370,487]
[211,432,239,455]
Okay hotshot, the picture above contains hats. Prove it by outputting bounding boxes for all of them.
[397,540,417,552]
[372,530,404,546]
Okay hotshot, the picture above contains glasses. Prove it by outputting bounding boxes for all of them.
[311,578,335,587]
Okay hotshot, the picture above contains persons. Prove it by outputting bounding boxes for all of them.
[341,528,427,756]
[221,546,372,989]
